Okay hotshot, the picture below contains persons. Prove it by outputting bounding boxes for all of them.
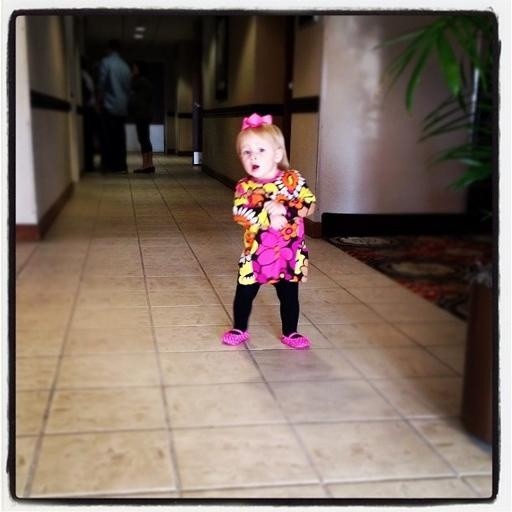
[129,60,156,174]
[223,111,317,349]
[82,60,102,173]
[95,38,134,173]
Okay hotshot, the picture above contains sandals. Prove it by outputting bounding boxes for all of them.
[222,328,250,346]
[278,331,312,350]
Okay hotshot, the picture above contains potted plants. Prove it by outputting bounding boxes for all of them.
[375,11,492,448]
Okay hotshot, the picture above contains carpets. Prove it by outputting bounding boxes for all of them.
[323,233,468,321]
